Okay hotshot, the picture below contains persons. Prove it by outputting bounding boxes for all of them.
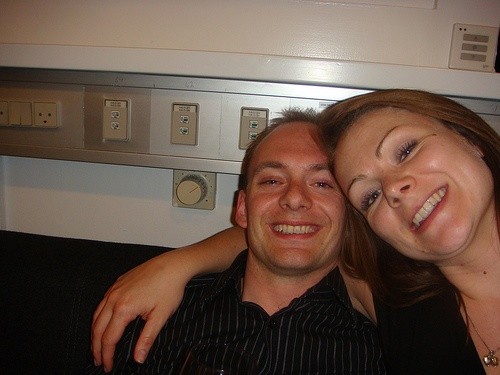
[91,89,500,375]
[82,113,387,375]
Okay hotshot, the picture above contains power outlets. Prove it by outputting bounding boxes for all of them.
[238,106,271,151]
[0,99,9,127]
[102,94,132,145]
[32,100,62,130]
[170,103,199,147]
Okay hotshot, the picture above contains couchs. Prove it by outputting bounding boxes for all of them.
[0,227,177,375]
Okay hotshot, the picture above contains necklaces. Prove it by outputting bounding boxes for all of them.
[468,316,500,368]
